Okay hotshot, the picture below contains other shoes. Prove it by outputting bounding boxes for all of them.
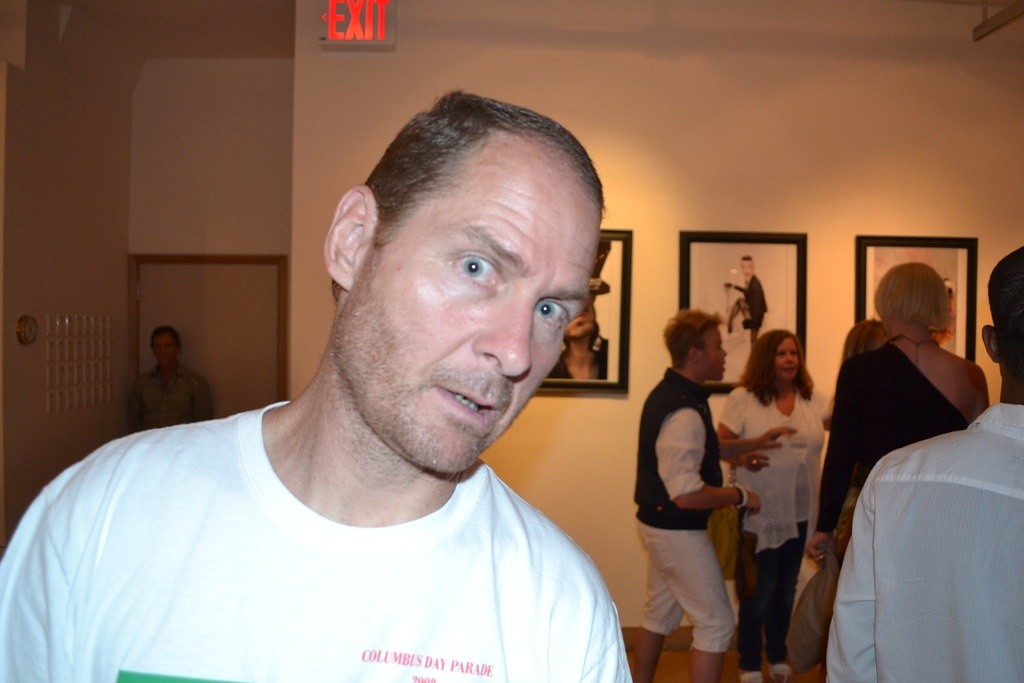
[770,662,791,682]
[738,669,766,683]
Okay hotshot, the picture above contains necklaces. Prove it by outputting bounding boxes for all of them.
[892,330,938,367]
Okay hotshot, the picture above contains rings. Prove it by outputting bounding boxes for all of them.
[750,458,759,465]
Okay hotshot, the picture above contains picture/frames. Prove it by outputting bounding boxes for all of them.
[677,231,807,393]
[852,234,979,367]
[535,229,634,393]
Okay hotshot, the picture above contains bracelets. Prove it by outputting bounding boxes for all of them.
[733,483,750,509]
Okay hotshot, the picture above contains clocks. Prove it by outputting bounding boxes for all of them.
[16,314,40,345]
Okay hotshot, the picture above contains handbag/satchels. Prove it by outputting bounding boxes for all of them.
[785,546,839,672]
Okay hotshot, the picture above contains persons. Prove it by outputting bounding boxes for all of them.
[721,254,770,354]
[824,242,1024,683]
[122,325,215,437]
[716,328,837,683]
[805,260,991,570]
[2,91,636,683]
[633,308,797,683]
[843,319,889,361]
